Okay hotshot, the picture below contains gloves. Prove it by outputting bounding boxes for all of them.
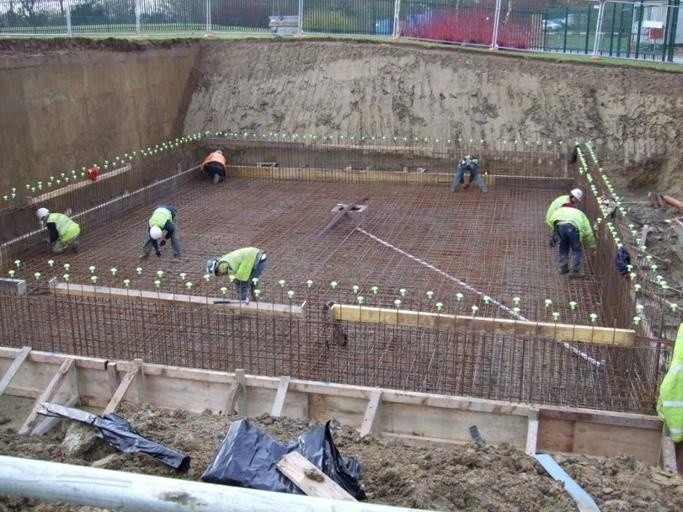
[160,240,165,246]
[156,251,161,258]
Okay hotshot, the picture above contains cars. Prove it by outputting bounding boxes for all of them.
[543,18,565,31]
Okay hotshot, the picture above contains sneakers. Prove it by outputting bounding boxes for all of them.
[72,241,80,253]
[213,173,219,185]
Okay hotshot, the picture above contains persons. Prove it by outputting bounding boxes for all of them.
[138,204,181,264]
[199,150,226,185]
[36,208,81,254]
[450,154,489,194]
[546,188,583,248]
[549,207,598,277]
[205,246,267,306]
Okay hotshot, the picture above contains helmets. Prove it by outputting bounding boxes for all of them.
[570,187,583,202]
[149,225,162,240]
[206,259,219,277]
[37,207,48,220]
